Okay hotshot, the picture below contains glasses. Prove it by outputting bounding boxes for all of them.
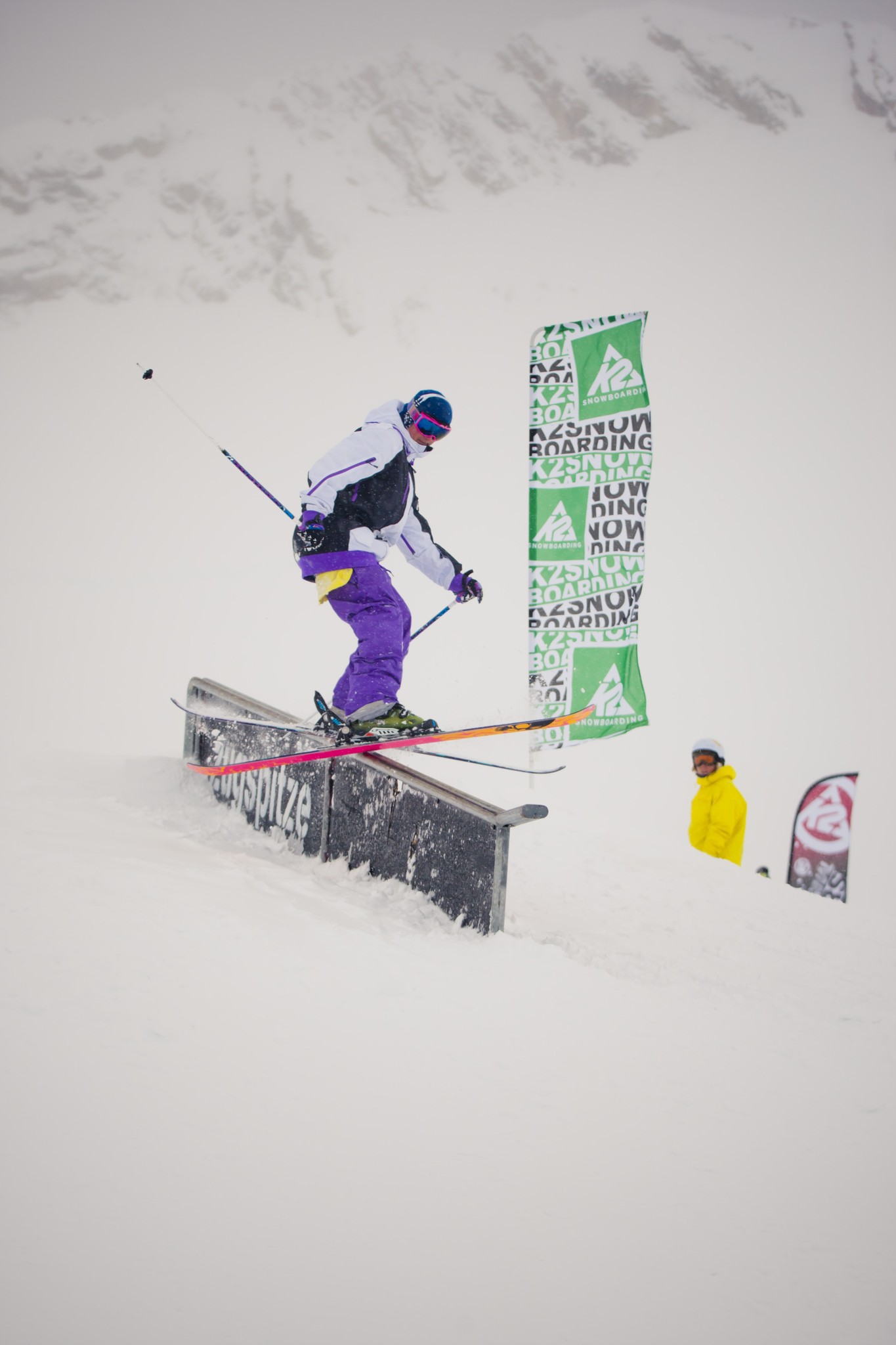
[407,397,452,441]
[693,753,718,766]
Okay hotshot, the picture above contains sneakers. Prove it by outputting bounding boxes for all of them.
[344,703,425,737]
[319,706,346,738]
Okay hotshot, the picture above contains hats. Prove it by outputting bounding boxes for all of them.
[402,389,452,429]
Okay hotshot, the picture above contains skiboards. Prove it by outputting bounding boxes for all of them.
[171,696,596,777]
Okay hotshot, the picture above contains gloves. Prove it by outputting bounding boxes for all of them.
[294,509,325,553]
[449,573,483,604]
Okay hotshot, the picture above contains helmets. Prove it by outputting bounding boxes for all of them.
[691,738,725,768]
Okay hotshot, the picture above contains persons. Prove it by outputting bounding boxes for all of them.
[681,738,747,871]
[288,385,484,736]
